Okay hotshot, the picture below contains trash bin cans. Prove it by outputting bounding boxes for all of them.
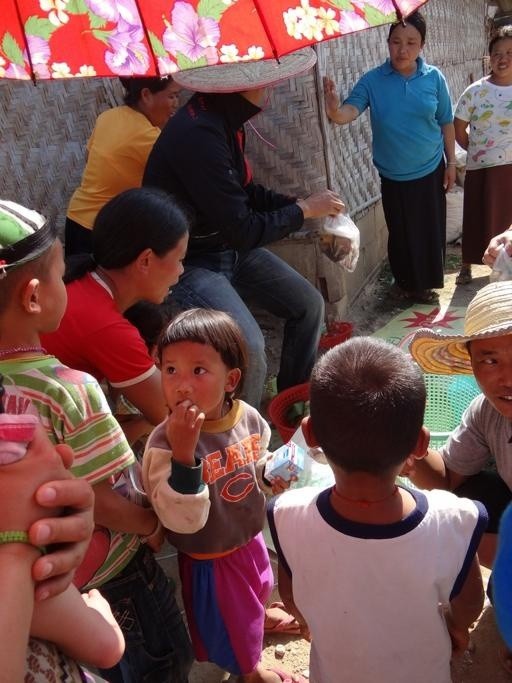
[395,372,483,491]
[267,383,320,448]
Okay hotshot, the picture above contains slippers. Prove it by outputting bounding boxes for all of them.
[255,665,303,683]
[261,601,302,634]
[454,265,475,283]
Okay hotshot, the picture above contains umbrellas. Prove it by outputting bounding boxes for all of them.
[0,1,428,86]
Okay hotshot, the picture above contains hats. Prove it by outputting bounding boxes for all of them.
[167,45,318,95]
[0,198,58,274]
[408,279,512,375]
